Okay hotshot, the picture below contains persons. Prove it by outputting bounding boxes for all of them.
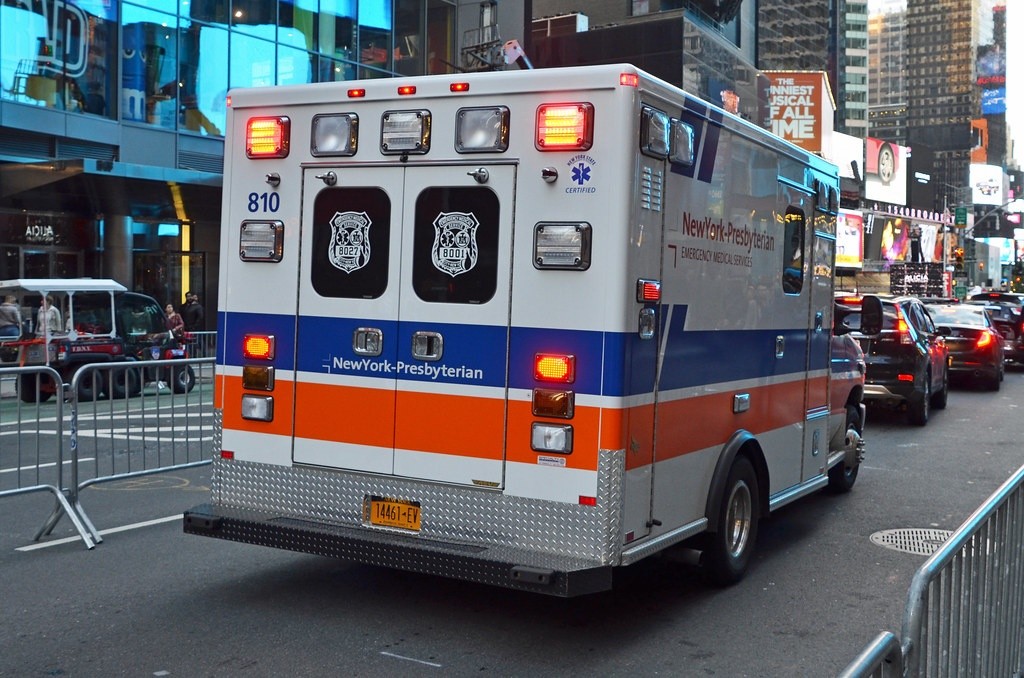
[181,292,207,357]
[34,296,61,338]
[0,295,21,336]
[165,303,184,334]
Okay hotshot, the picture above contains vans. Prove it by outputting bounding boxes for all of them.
[16,291,196,403]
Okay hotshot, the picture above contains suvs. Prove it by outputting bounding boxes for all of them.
[832,294,951,428]
[971,293,1022,314]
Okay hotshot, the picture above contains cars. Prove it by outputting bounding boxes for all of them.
[923,303,1003,393]
[987,304,1024,367]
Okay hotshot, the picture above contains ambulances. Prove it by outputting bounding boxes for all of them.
[183,61,885,597]
